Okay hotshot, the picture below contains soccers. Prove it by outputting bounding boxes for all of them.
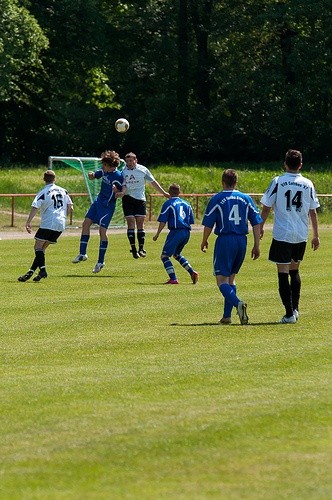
[115,118,129,133]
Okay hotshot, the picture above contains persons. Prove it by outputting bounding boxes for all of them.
[153,183,199,286]
[260,149,321,325]
[200,168,264,325]
[17,170,75,282]
[71,150,127,274]
[114,152,171,259]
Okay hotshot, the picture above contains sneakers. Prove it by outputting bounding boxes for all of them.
[18,272,32,282]
[218,317,231,324]
[92,262,105,273]
[293,309,298,320]
[277,315,296,324]
[236,301,248,325]
[191,271,198,284]
[138,250,147,257]
[33,272,47,281]
[72,254,87,263]
[164,279,178,284]
[132,252,140,258]
